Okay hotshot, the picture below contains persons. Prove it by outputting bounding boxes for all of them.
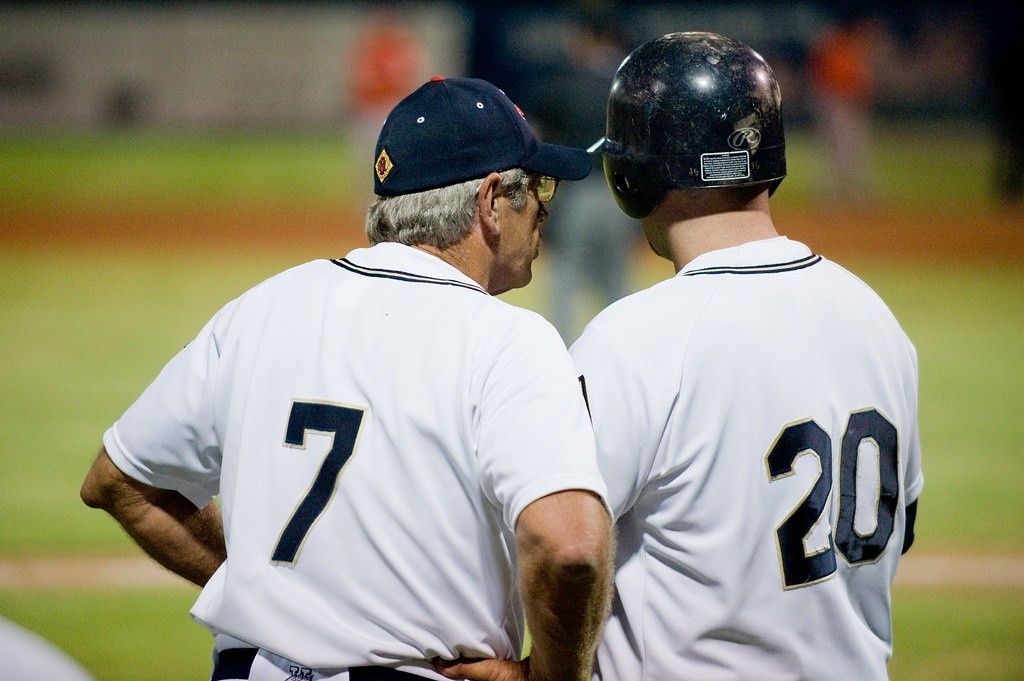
[79,74,614,681]
[355,0,1023,369]
[563,30,925,681]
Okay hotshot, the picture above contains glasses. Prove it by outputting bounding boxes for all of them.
[519,172,561,202]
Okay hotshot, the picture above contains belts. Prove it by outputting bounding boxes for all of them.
[209,647,429,681]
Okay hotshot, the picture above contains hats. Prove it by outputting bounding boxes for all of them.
[373,76,592,196]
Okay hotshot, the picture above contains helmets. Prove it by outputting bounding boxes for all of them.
[588,32,786,219]
